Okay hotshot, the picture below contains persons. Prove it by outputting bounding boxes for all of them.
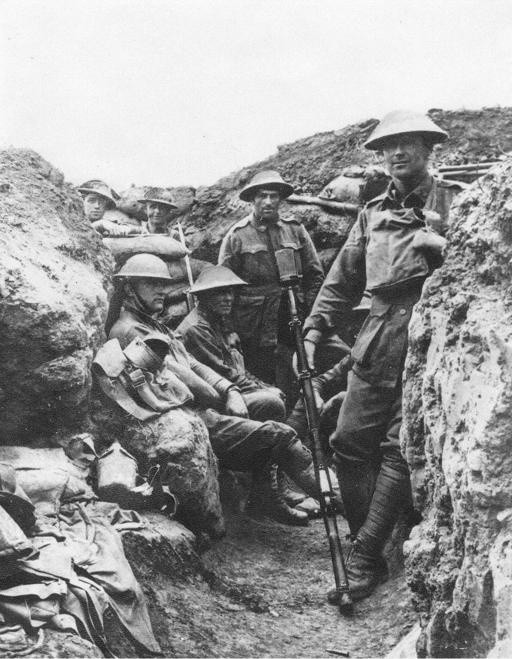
[292,108,468,606]
[136,186,178,235]
[108,254,348,520]
[285,297,373,437]
[219,170,328,381]
[79,179,138,238]
[173,263,310,526]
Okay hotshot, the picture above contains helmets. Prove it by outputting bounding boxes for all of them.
[365,110,447,148]
[137,187,179,208]
[190,266,249,293]
[240,170,293,201]
[76,186,118,208]
[115,253,172,281]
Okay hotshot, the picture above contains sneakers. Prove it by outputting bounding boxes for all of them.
[329,541,389,601]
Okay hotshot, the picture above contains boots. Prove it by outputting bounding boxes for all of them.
[245,439,345,526]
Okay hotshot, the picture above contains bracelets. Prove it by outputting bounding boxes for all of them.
[302,331,319,346]
